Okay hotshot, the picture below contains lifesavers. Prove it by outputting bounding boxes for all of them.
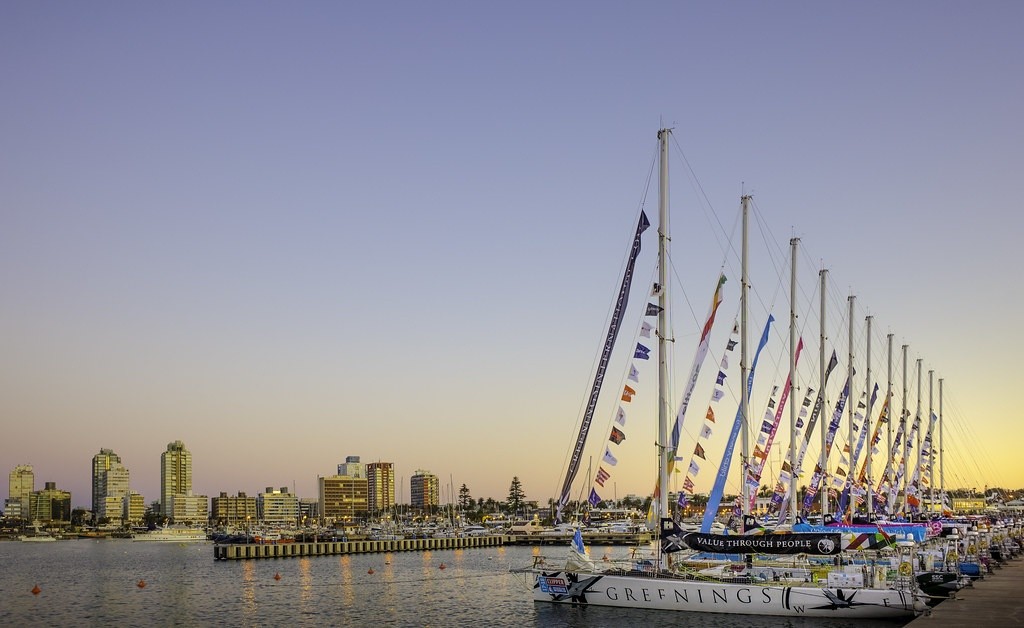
[898,562,912,576]
[977,528,1024,548]
[968,544,977,555]
[946,551,958,562]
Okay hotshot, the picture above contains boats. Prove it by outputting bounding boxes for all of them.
[9,521,149,543]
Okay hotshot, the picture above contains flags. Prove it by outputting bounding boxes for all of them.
[677,319,739,508]
[570,528,585,555]
[828,401,865,498]
[732,386,778,518]
[767,386,815,518]
[573,282,666,528]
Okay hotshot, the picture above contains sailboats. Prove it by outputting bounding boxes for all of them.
[504,106,1023,620]
[202,479,491,542]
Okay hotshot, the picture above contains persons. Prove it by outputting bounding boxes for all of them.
[834,555,855,566]
